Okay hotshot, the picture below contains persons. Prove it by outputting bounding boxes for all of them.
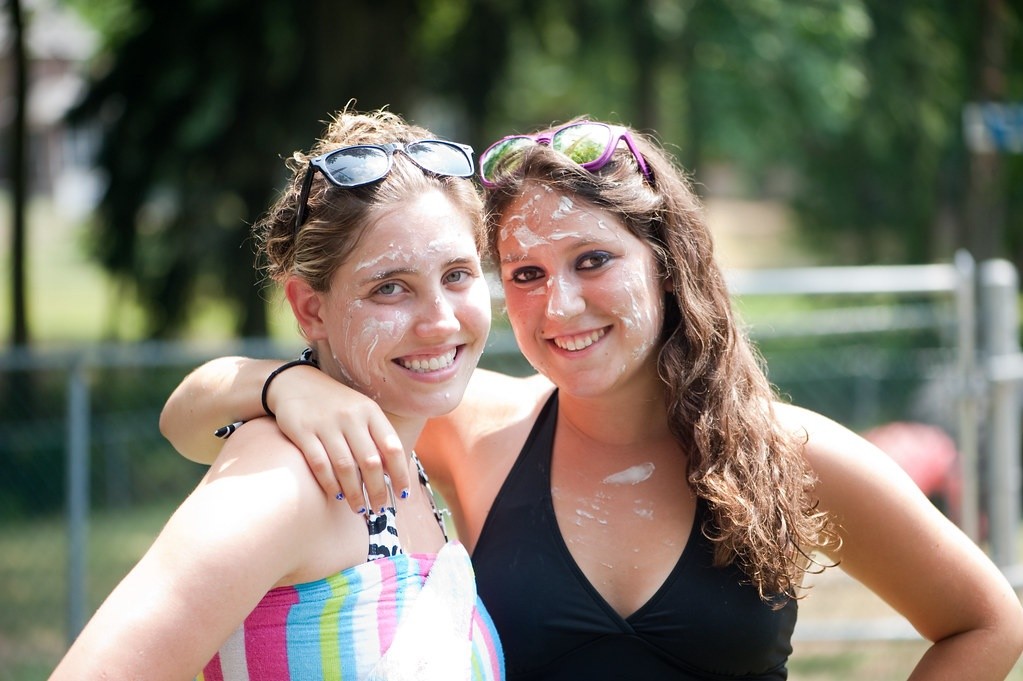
[159,114,1022,681]
[45,96,505,681]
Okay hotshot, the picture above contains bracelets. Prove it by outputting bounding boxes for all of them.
[263,360,320,418]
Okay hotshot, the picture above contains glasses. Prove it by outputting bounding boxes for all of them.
[480,121,655,201]
[292,138,474,248]
